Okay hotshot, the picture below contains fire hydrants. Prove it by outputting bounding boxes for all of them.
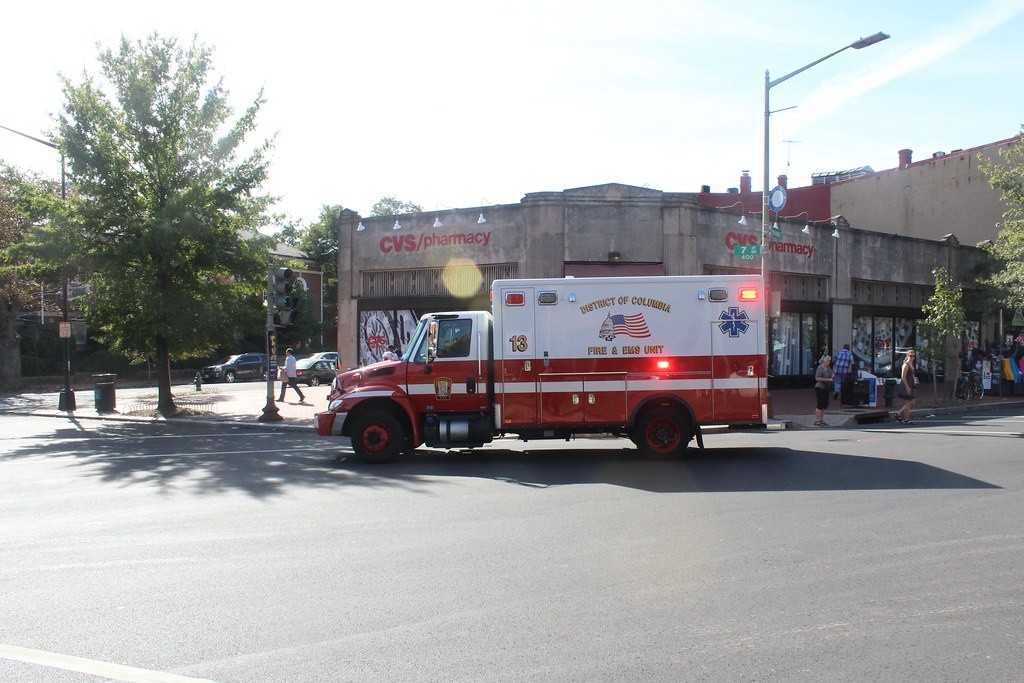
[882,371,897,407]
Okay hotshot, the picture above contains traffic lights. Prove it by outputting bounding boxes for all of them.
[275,267,293,309]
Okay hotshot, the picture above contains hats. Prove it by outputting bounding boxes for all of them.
[959,351,965,359]
[990,354,997,358]
[1015,336,1024,343]
[1019,340,1024,346]
[997,355,1004,362]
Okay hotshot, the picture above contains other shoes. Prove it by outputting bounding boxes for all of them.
[300,396,305,401]
[833,392,839,399]
[904,420,915,425]
[276,399,284,402]
[894,413,902,423]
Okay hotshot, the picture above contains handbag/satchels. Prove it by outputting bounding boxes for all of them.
[993,365,1000,373]
[822,377,835,391]
[277,368,289,383]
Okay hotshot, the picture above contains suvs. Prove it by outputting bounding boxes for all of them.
[201,353,268,383]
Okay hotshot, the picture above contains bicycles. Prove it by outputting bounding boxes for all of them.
[959,372,984,403]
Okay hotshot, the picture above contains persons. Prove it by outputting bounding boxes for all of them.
[275,348,305,402]
[382,345,401,361]
[894,350,915,425]
[833,344,854,399]
[814,355,835,426]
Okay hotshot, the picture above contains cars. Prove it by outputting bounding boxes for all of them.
[310,352,338,365]
[290,359,335,387]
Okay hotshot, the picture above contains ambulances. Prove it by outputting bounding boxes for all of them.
[314,275,768,465]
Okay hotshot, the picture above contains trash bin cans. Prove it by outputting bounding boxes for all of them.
[91,372,119,410]
[840,379,870,408]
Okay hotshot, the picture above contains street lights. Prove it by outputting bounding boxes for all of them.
[763,32,890,407]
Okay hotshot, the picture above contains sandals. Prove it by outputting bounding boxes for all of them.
[814,420,828,427]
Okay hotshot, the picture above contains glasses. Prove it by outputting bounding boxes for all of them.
[384,358,388,360]
[908,355,916,357]
